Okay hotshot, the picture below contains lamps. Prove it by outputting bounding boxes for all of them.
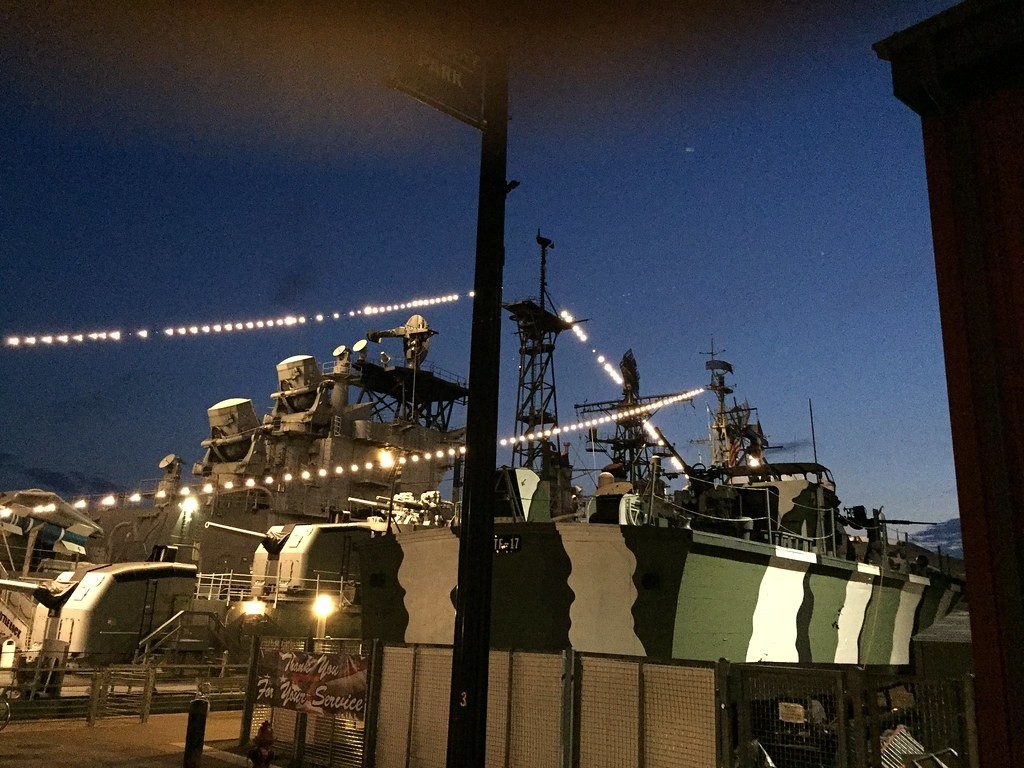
[331,339,368,363]
